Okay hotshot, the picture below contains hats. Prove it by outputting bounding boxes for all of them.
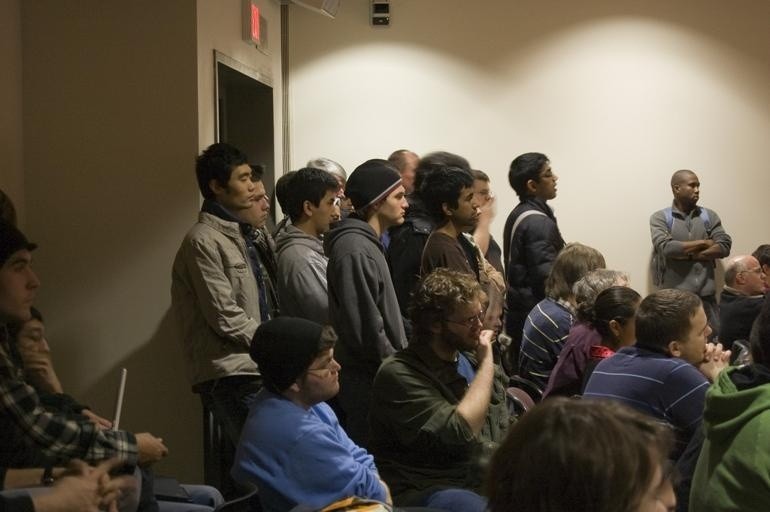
[345,158,402,211]
[249,317,327,394]
[0,222,37,266]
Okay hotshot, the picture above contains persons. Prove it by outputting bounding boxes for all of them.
[471,168,503,273]
[170,142,280,450]
[718,255,766,351]
[306,157,346,213]
[751,244,770,291]
[541,269,628,399]
[0,224,225,510]
[10,304,112,429]
[518,242,606,389]
[275,171,295,219]
[419,165,480,283]
[580,286,643,393]
[489,395,677,512]
[583,289,731,463]
[386,152,472,279]
[276,167,340,313]
[0,454,137,511]
[387,148,421,195]
[502,151,564,341]
[648,169,732,307]
[231,164,269,228]
[231,316,393,512]
[369,266,509,512]
[687,312,770,512]
[324,159,409,367]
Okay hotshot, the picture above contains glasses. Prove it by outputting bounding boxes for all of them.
[445,311,485,327]
